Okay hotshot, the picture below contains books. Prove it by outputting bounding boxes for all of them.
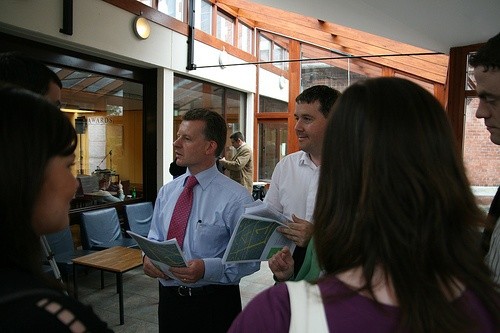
[126,230,190,285]
[221,198,298,263]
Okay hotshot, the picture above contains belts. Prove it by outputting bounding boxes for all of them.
[160,284,232,297]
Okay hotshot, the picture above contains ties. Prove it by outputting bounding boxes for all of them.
[166,176,199,250]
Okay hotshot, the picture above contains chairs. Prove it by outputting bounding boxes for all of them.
[42,194,154,298]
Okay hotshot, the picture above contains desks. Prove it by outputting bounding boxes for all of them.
[85,191,116,199]
[72,246,144,325]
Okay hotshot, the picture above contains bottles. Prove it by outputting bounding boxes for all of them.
[132,187,137,198]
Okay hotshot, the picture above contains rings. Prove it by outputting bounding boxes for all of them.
[183,278,187,281]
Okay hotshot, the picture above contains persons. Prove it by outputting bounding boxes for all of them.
[225,76,500,333]
[143,109,262,333]
[263,84,341,295]
[99,172,125,204]
[0,54,63,110]
[0,84,115,333]
[470,33,500,285]
[169,158,187,180]
[219,131,253,195]
